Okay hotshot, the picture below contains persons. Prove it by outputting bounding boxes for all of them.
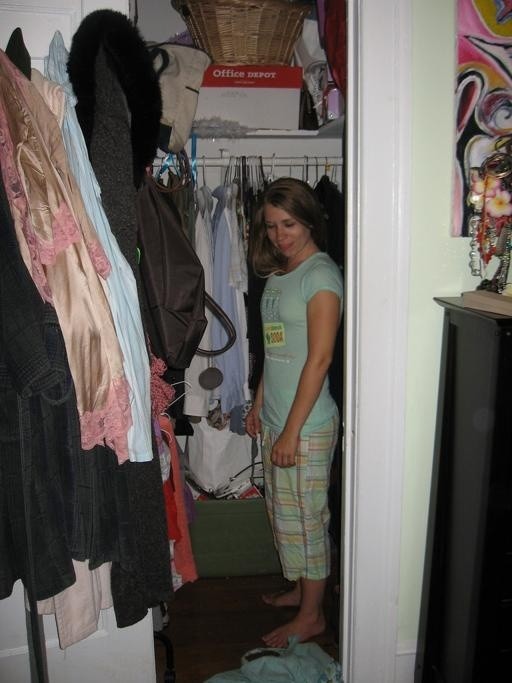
[246,177,342,647]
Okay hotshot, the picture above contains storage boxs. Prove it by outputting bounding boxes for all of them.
[189,65,303,139]
[189,486,282,578]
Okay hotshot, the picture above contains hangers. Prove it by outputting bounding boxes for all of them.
[0,587,175,683]
[146,153,344,189]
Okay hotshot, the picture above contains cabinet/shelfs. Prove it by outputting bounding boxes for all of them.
[415,296,511,682]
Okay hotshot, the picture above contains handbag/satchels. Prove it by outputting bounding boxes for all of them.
[240,634,342,683]
[145,43,212,155]
[135,166,235,370]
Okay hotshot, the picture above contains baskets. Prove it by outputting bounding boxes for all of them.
[171,0,313,67]
